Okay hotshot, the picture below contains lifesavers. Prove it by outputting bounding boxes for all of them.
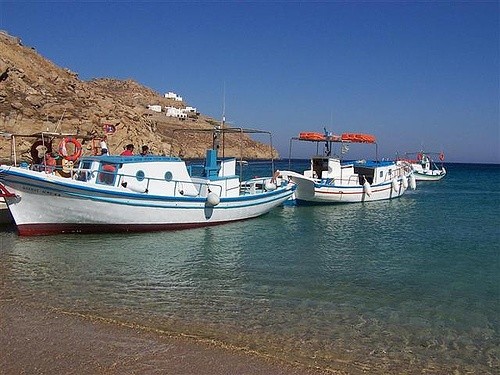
[56,156,80,178]
[31,141,51,164]
[58,137,82,162]
[439,153,445,161]
[416,153,423,160]
[99,165,116,181]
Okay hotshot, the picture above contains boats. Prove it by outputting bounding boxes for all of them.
[0,120,299,239]
[281,131,415,202]
[402,153,446,181]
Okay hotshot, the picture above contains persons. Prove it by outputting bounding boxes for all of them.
[214,136,221,157]
[139,145,148,155]
[120,144,135,155]
